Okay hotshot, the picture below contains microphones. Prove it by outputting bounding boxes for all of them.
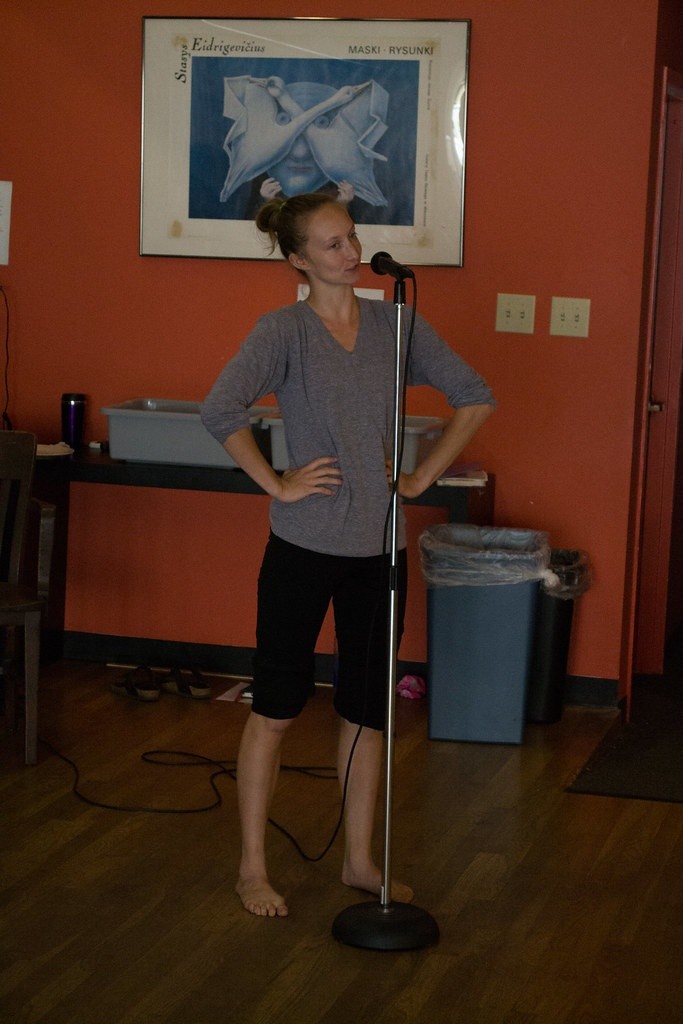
[370,250,415,278]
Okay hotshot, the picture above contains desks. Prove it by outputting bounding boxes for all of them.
[34,443,495,685]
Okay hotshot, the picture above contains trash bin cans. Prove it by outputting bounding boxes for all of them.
[528,548,590,724]
[421,524,548,744]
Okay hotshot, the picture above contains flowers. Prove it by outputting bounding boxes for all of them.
[396,675,425,699]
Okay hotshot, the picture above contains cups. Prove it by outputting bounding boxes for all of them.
[61,393,86,453]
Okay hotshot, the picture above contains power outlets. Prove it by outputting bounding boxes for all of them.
[494,293,536,335]
[549,297,591,338]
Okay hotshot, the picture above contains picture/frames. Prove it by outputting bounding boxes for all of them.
[140,15,470,269]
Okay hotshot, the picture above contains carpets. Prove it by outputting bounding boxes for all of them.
[562,616,683,803]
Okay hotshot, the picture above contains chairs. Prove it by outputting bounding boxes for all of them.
[0,432,56,766]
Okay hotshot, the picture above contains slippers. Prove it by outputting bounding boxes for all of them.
[111,666,160,701]
[164,665,211,698]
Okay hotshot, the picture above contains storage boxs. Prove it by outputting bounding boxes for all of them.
[100,399,274,469]
[261,414,445,475]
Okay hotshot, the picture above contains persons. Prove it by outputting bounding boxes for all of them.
[198,194,498,918]
